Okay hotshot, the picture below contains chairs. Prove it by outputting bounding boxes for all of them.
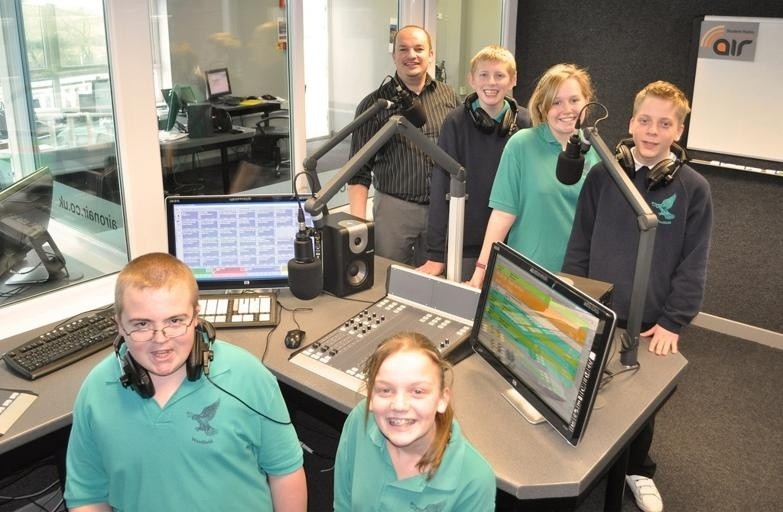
[255,111,291,177]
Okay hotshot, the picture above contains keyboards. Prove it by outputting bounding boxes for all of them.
[2,313,120,381]
[187,293,278,329]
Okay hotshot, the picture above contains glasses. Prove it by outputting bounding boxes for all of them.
[113,312,199,340]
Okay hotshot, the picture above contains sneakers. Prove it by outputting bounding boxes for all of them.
[626,472,664,512]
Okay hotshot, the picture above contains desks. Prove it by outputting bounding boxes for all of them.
[209,98,283,128]
[161,125,257,195]
[0,256,687,511]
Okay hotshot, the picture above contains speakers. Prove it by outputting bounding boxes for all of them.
[188,103,214,138]
[314,212,375,298]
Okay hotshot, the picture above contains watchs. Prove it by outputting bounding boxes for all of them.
[475,261,487,271]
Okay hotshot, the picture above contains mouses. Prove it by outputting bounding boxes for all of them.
[247,95,257,99]
[285,330,306,348]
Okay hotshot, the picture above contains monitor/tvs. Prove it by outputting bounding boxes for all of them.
[2,167,67,286]
[206,68,232,101]
[168,83,187,133]
[470,242,617,448]
[161,86,197,113]
[165,195,322,295]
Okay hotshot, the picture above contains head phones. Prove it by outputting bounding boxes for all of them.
[113,318,216,399]
[465,93,521,138]
[614,138,689,193]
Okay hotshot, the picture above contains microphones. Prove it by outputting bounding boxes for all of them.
[287,170,325,300]
[555,103,609,185]
[377,73,432,133]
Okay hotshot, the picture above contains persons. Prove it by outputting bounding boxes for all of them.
[348,25,465,267]
[411,45,537,285]
[332,334,498,511]
[561,81,713,511]
[64,253,310,511]
[467,63,608,292]
